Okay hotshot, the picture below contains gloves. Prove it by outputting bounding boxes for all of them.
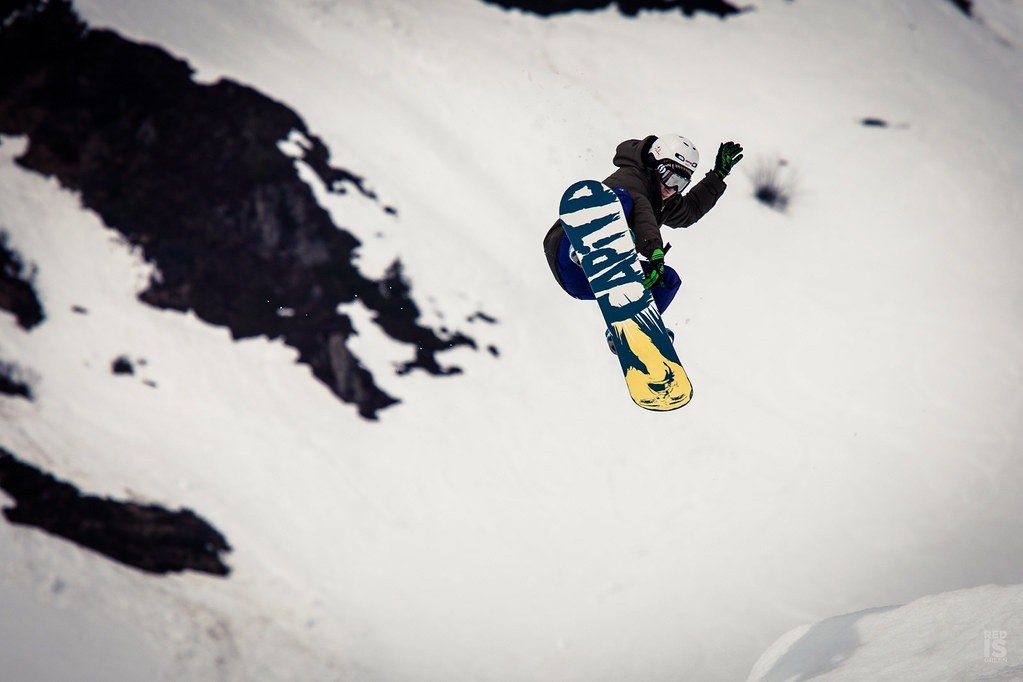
[714,141,743,177]
[642,250,664,290]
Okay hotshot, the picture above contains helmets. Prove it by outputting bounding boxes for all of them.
[648,135,699,177]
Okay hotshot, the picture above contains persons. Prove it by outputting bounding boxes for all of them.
[542,135,744,355]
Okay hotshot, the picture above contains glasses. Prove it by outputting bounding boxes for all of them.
[658,167,690,194]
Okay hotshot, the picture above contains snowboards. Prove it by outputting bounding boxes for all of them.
[558,180,693,411]
